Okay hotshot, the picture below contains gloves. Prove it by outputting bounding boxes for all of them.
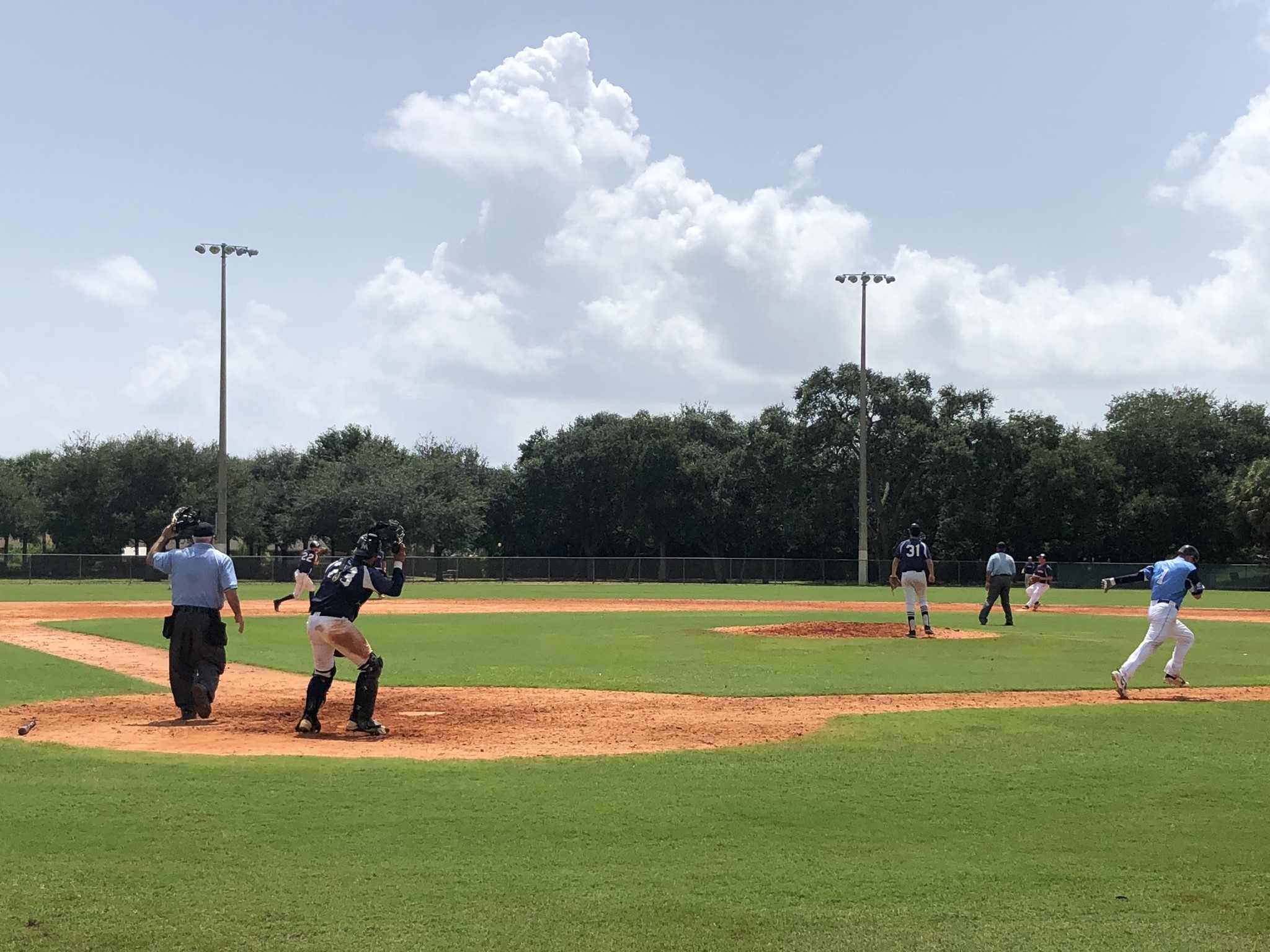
[1101,577,1116,593]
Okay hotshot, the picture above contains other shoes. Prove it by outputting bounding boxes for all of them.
[181,684,212,720]
[379,595,385,599]
[1005,622,1013,626]
[273,599,281,612]
[979,614,986,625]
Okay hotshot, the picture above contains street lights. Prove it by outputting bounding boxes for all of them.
[195,243,259,554]
[835,272,900,586]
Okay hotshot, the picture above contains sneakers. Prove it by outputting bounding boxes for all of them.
[299,714,321,733]
[924,625,934,635]
[345,712,390,737]
[1111,668,1127,698]
[1021,605,1029,609]
[1163,677,1189,687]
[1032,602,1041,611]
[905,630,916,638]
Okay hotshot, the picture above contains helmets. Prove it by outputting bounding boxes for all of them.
[1177,545,1199,563]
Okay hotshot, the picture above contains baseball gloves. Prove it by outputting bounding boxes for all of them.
[888,575,902,596]
[1031,575,1040,583]
[316,547,328,556]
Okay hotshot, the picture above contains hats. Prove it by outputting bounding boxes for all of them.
[193,522,215,537]
[907,523,920,530]
[1036,553,1046,558]
[310,541,322,548]
[353,533,380,558]
[996,542,1006,547]
[1028,556,1032,560]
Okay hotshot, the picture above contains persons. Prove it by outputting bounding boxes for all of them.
[1022,553,1054,611]
[273,540,329,613]
[891,522,935,638]
[146,504,245,720]
[295,532,406,736]
[1021,556,1037,588]
[368,524,387,599]
[1100,544,1206,700]
[978,541,1017,626]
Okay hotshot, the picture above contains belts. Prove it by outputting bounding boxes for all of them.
[1157,600,1179,611]
[310,612,342,618]
[991,574,1010,578]
